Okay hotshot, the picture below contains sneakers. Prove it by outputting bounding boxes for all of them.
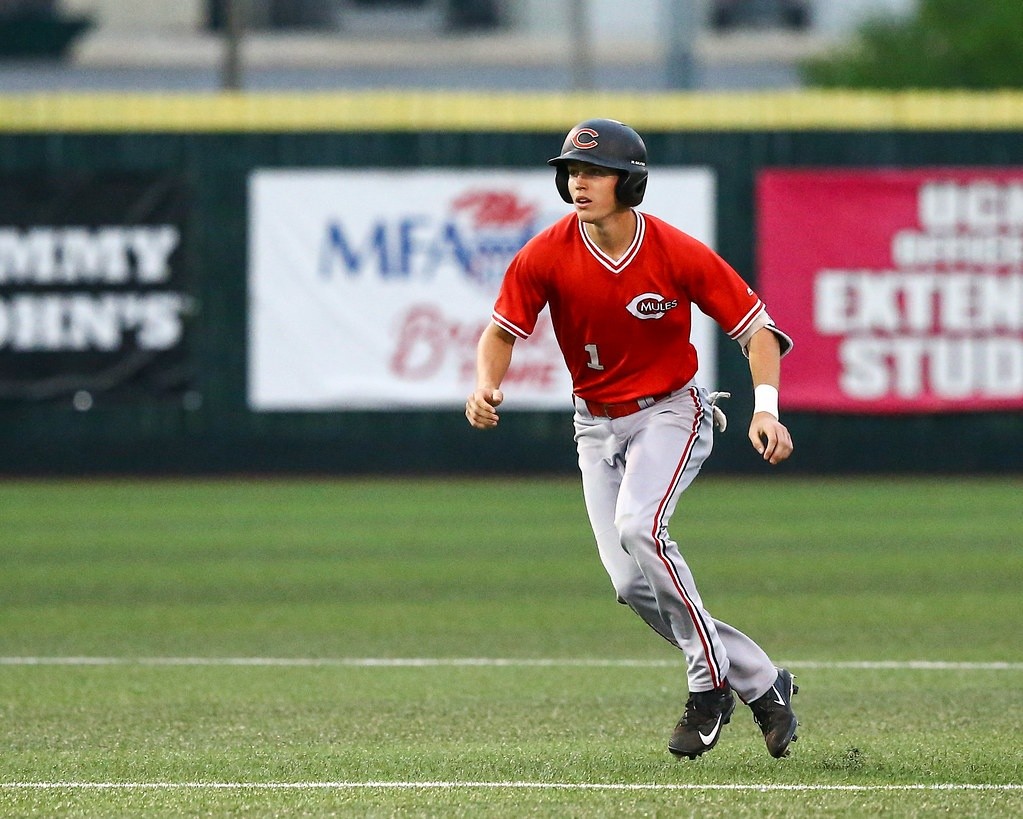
[668,676,736,759]
[747,668,802,758]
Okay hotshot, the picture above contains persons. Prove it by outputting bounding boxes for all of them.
[465,117,801,760]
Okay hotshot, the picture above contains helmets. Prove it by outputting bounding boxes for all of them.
[547,118,648,207]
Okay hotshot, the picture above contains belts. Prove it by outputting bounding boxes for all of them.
[572,394,671,420]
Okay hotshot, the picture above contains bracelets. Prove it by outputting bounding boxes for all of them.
[754,384,779,421]
